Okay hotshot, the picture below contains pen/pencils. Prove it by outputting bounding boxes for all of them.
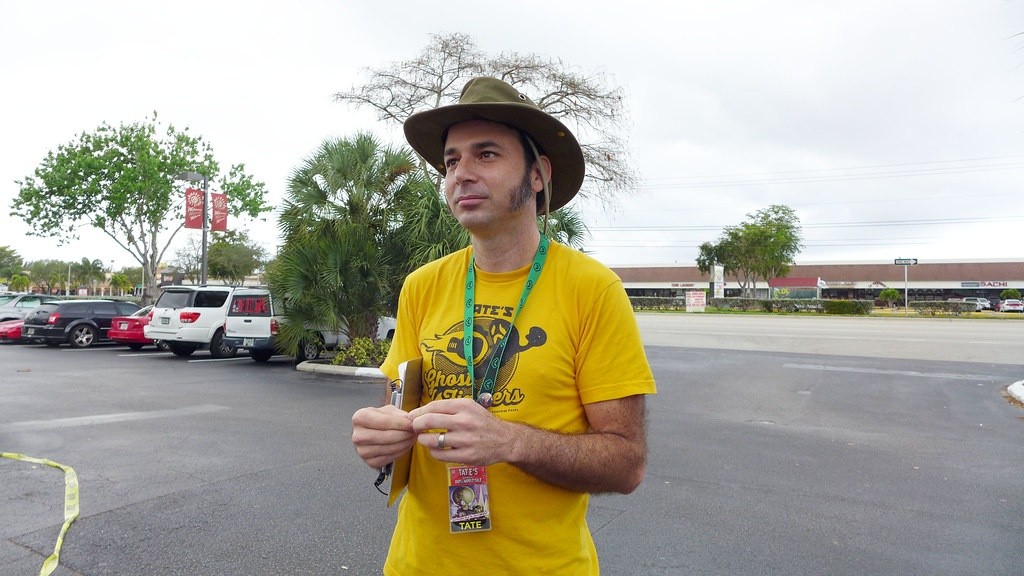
[381,390,403,482]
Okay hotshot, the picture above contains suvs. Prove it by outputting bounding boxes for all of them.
[142,284,256,358]
[223,283,398,361]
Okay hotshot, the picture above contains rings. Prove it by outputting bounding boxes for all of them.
[438,432,445,448]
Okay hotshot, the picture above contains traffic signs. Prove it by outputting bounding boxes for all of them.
[894,259,918,265]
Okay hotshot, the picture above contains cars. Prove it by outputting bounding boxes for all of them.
[106,304,171,352]
[0,292,65,342]
[20,298,143,349]
[963,297,983,312]
[978,297,991,310]
[999,299,1024,312]
[0,319,24,342]
[993,300,1004,313]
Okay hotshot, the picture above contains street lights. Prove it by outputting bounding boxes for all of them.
[177,170,210,287]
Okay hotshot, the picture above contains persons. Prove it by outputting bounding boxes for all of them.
[352,77,657,576]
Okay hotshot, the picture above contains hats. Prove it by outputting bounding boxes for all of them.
[403,76,585,216]
